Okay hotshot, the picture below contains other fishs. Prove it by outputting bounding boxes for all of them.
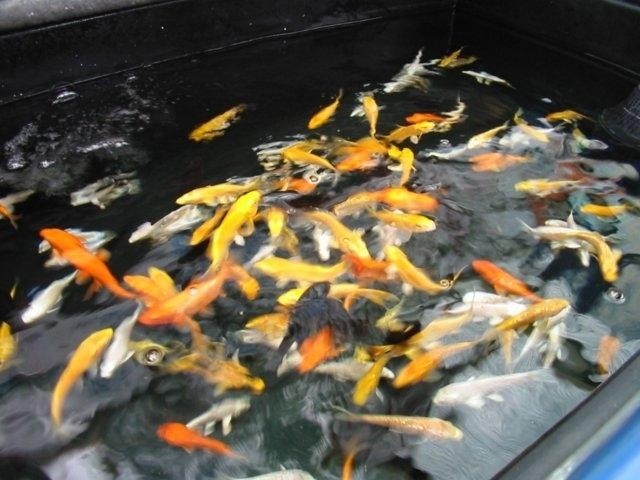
[0,89,635,461]
